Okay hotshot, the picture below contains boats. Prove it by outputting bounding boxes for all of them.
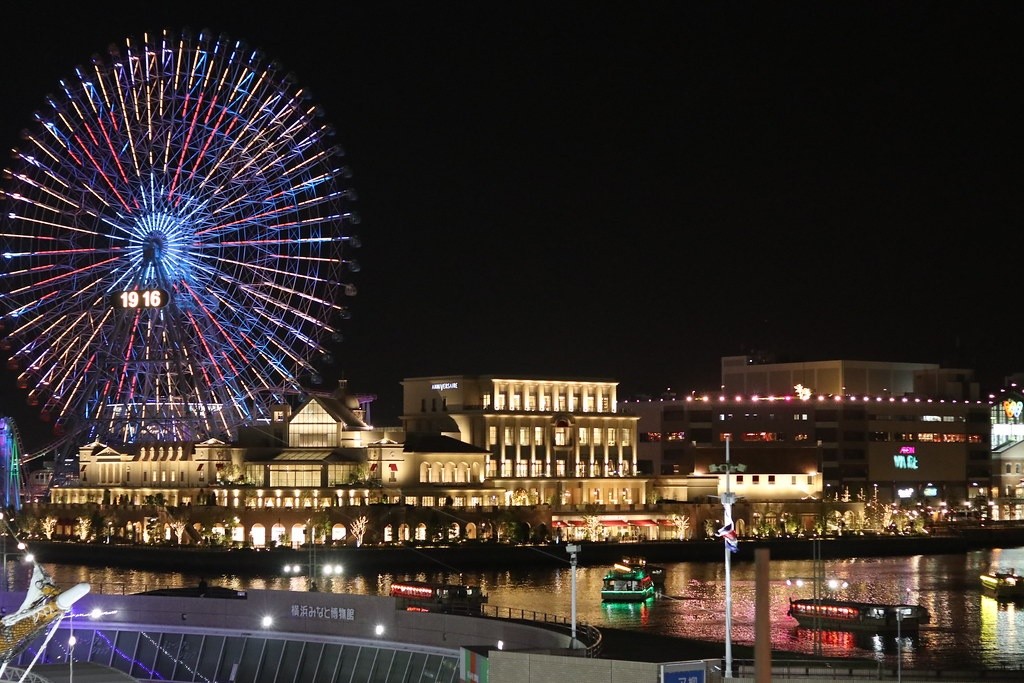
[787,598,932,632]
[601,556,666,601]
[389,580,489,616]
[980,568,1024,590]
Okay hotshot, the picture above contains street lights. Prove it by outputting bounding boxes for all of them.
[720,433,737,679]
